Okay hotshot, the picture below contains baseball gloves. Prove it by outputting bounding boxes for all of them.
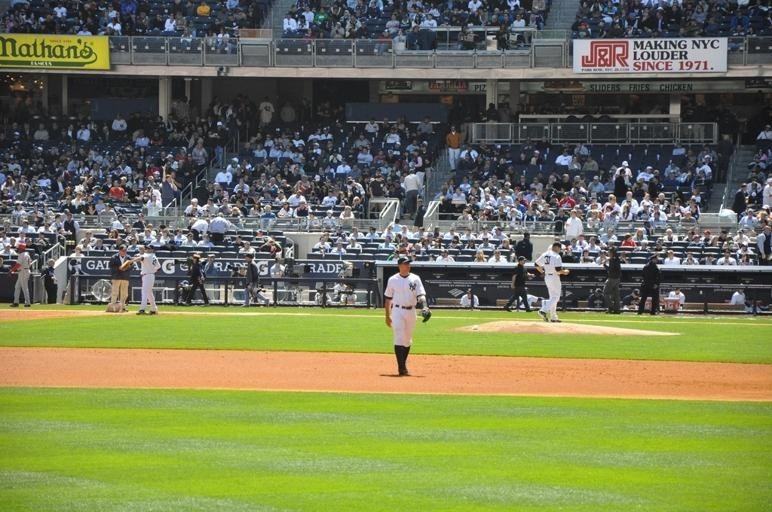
[421,311,432,323]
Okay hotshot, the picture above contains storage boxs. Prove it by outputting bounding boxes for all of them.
[663,297,680,310]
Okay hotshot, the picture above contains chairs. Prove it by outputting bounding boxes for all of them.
[668,0,752,37]
[365,3,395,39]
[144,1,224,54]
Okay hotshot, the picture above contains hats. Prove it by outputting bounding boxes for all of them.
[518,255,528,262]
[15,242,28,251]
[761,204,769,210]
[647,253,658,260]
[397,256,413,266]
[621,161,630,167]
[145,242,155,251]
[552,240,565,250]
[242,252,254,258]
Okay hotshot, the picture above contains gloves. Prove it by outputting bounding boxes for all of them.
[421,306,431,323]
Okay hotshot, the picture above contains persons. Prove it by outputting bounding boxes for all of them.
[384,255,430,376]
[1,1,771,323]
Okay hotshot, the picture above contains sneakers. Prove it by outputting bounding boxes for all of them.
[550,317,563,323]
[398,366,410,375]
[240,303,250,308]
[181,301,211,307]
[265,298,270,309]
[9,302,20,308]
[538,310,549,323]
[23,303,31,308]
[135,309,146,315]
[503,305,513,313]
[145,310,158,315]
[525,307,535,313]
